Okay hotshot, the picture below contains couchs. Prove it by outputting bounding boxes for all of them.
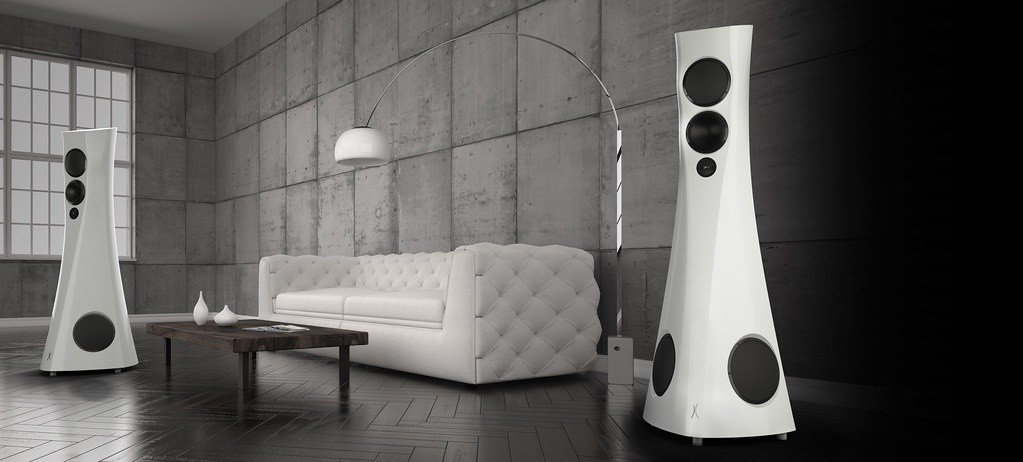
[258,242,602,388]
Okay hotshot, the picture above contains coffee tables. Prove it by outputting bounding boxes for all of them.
[147,320,369,392]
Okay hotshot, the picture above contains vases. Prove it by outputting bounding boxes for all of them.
[193,291,209,325]
[213,305,238,326]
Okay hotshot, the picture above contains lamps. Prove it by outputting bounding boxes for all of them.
[334,31,634,385]
